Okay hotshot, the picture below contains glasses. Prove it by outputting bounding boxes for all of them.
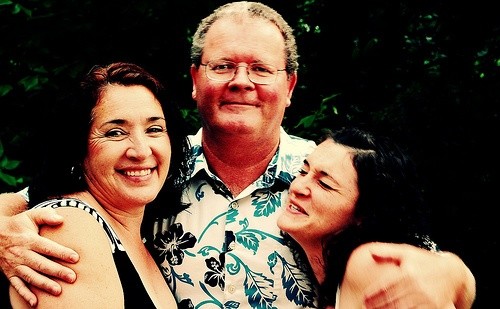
[198,59,292,85]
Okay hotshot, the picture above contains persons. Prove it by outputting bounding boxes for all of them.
[276,126,457,309]
[0,62,193,309]
[0,1,476,309]
